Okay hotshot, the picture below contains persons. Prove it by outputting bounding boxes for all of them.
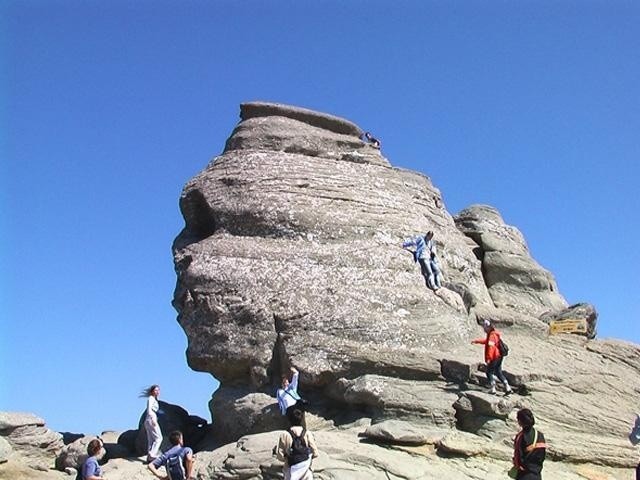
[273,408,318,480]
[275,366,306,418]
[148,431,194,480]
[401,229,441,291]
[137,384,164,464]
[469,318,515,396]
[78,439,106,479]
[365,131,382,150]
[511,408,546,480]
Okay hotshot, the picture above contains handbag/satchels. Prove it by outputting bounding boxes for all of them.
[497,340,509,356]
[297,398,312,411]
[507,467,523,480]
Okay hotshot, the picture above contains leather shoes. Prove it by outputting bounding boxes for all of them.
[503,390,514,397]
[488,390,497,396]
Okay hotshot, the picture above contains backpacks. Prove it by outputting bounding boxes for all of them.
[287,427,309,466]
[162,447,187,480]
